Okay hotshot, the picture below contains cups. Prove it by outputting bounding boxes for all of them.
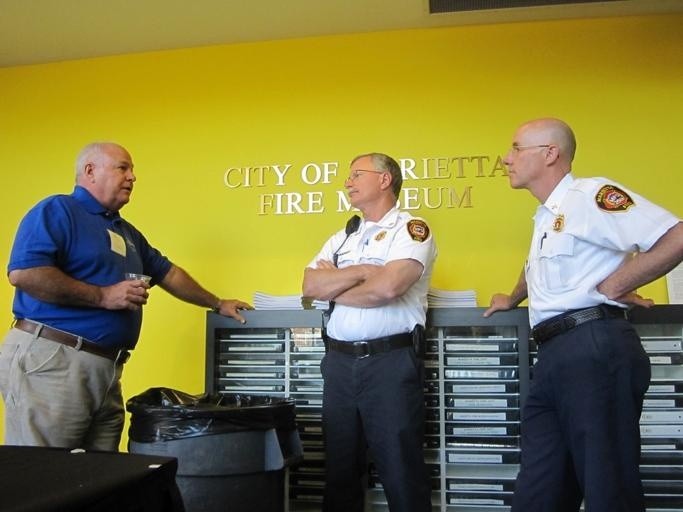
[125,273,152,310]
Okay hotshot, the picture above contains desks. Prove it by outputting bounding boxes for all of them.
[0,445,186,512]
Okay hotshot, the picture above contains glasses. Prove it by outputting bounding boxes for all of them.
[508,143,560,159]
[345,170,388,185]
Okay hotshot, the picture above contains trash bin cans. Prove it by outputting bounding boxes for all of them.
[127,387,304,510]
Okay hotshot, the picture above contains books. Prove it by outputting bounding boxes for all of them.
[426,286,477,308]
[253,290,331,311]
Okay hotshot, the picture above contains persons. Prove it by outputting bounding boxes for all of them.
[0,141,256,453]
[299,152,438,512]
[483,117,683,512]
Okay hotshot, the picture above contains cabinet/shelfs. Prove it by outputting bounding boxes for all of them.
[206,306,683,511]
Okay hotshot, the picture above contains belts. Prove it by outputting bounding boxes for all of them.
[532,303,631,346]
[12,319,132,364]
[322,331,414,359]
